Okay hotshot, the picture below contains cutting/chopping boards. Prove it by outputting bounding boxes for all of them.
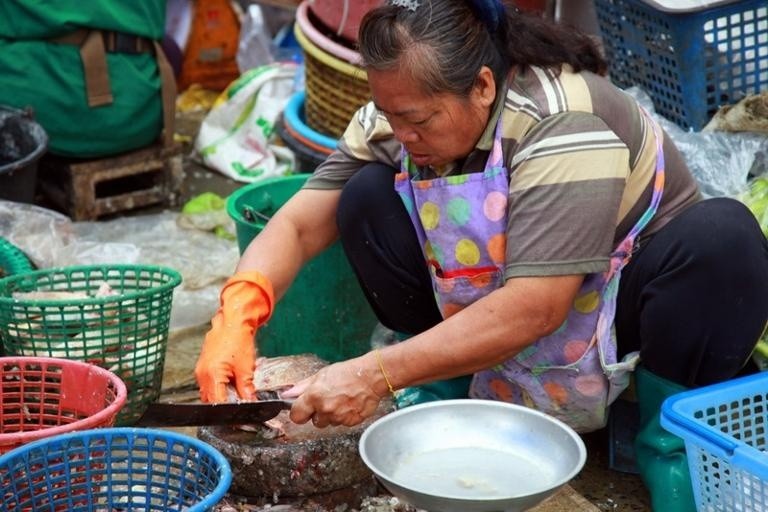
[199,353,396,497]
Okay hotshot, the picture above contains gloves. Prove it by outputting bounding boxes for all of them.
[195,271,274,404]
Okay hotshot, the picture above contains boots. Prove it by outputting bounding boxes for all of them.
[394,332,471,410]
[634,368,697,512]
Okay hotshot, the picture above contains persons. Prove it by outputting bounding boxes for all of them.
[0,0,178,159]
[193,0,768,512]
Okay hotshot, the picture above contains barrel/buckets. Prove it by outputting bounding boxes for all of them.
[227,172,376,366]
[0,101,48,204]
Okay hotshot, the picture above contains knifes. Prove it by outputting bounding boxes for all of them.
[137,402,295,424]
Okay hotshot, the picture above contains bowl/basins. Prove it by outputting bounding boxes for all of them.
[358,397,588,512]
[275,90,341,175]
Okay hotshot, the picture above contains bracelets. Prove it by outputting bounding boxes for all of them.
[375,349,394,394]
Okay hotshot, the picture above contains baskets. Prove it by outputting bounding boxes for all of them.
[293,21,371,139]
[0,356,127,512]
[659,371,767,512]
[0,262,182,425]
[0,262,234,512]
[596,1,768,132]
[0,427,232,512]
